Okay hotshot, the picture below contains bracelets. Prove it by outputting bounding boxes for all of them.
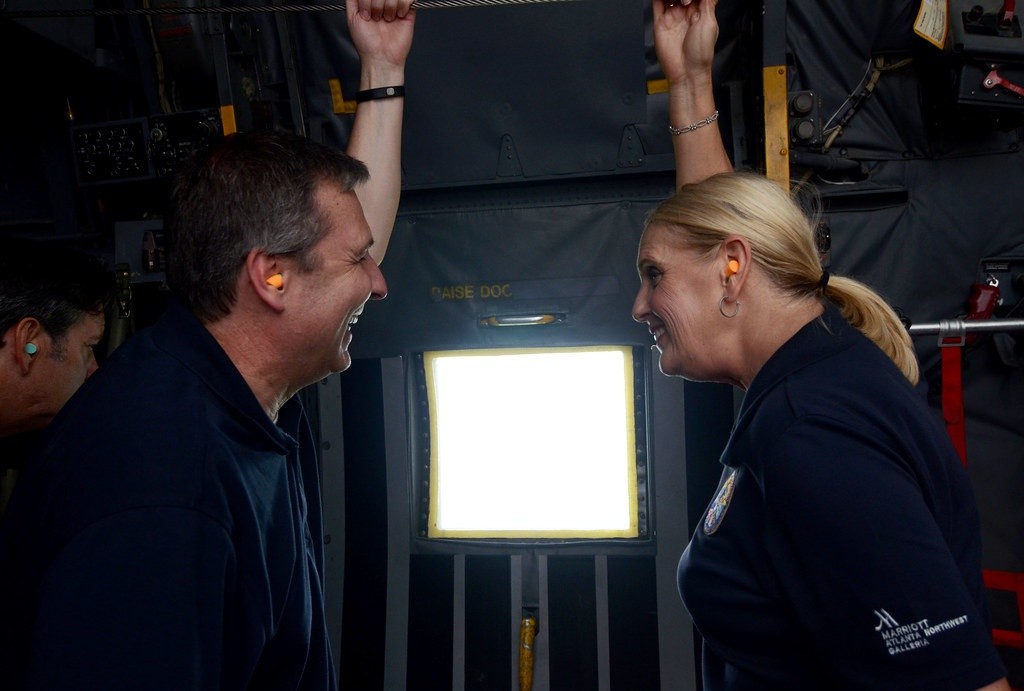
[356,86,406,104]
[669,110,718,135]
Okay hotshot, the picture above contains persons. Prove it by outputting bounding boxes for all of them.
[629,0,1014,691]
[0,237,117,438]
[0,0,416,691]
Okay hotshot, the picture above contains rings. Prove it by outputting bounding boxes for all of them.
[668,3,674,7]
